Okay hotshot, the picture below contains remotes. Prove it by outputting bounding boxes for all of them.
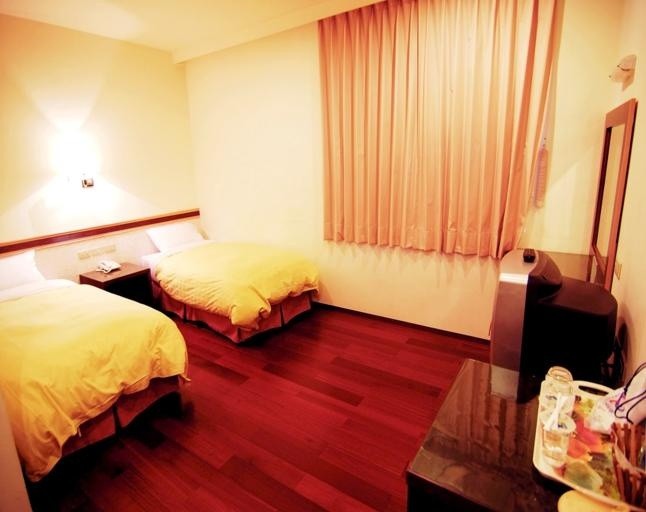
[524,249,535,263]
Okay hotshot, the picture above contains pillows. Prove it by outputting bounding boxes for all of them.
[0,249,43,292]
[144,219,206,257]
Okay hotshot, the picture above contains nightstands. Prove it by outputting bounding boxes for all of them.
[80,261,155,310]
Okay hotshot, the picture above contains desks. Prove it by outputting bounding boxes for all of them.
[404,359,630,511]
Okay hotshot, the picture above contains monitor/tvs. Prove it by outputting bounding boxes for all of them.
[488,248,617,404]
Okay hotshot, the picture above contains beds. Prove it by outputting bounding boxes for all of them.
[0,275,188,492]
[142,242,321,345]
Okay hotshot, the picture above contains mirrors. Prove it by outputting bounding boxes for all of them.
[583,98,636,295]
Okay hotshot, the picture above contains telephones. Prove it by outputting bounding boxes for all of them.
[97,260,121,271]
